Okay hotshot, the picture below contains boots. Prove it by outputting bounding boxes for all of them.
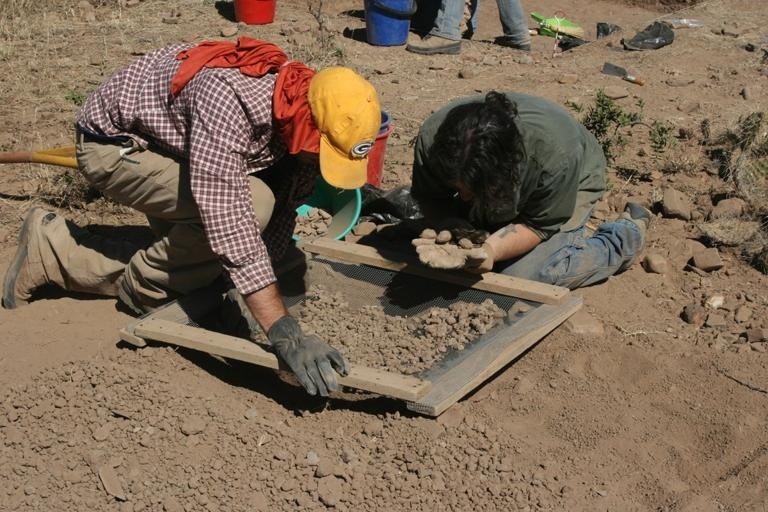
[493,36,531,50]
[116,273,167,317]
[3,207,55,309]
[406,32,461,54]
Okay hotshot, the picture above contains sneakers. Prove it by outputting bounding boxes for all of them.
[618,203,653,272]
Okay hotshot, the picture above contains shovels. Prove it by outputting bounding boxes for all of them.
[601,61,646,86]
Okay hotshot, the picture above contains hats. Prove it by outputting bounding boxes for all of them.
[308,67,381,190]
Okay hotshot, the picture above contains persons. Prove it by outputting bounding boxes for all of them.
[412,91,651,290]
[406,1,533,55]
[2,38,380,398]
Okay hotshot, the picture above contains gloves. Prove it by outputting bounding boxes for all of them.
[268,314,349,397]
[412,219,494,273]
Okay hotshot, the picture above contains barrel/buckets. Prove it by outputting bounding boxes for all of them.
[368,111,393,188]
[364,0,417,47]
[290,176,362,243]
[233,0,276,25]
